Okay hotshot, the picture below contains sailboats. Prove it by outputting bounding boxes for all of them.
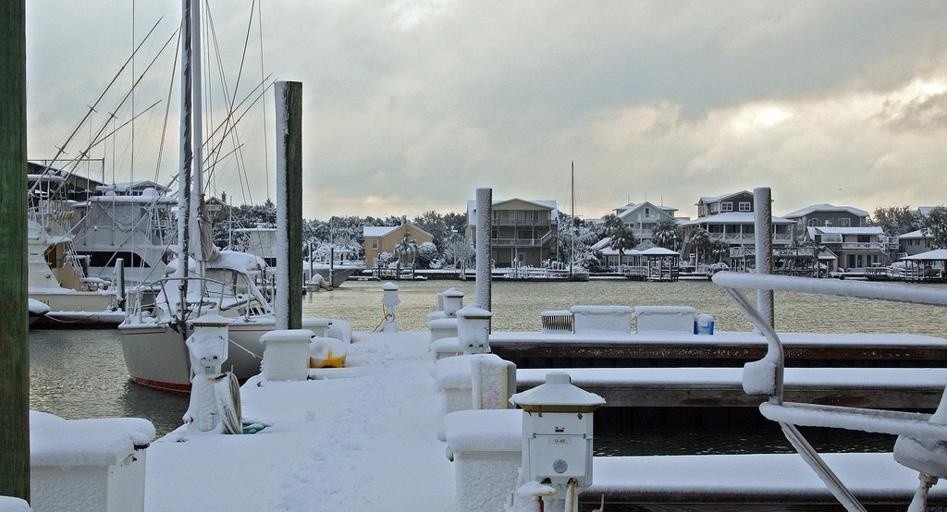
[117,0,329,400]
[27,0,265,330]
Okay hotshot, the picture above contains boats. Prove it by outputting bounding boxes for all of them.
[223,223,358,290]
